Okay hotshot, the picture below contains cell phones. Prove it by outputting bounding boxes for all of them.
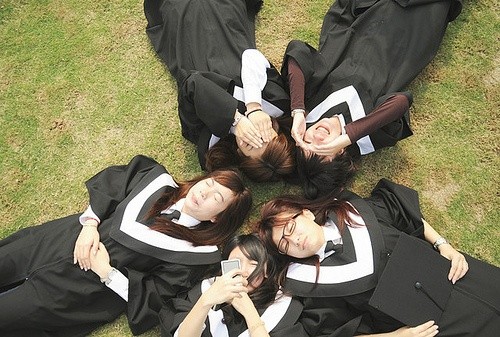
[221,258,244,287]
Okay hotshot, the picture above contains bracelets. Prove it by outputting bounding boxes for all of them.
[291,109,305,117]
[249,321,264,335]
[433,238,446,250]
[245,107,263,117]
[230,114,244,133]
[83,224,97,228]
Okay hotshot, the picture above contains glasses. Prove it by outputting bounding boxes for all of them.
[277,212,303,255]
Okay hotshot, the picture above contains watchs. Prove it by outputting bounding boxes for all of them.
[100,268,117,285]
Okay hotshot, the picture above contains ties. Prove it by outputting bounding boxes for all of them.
[237,100,247,114]
[159,210,180,221]
[324,241,343,253]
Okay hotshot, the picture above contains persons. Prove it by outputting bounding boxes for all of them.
[157,234,310,337]
[279,0,464,200]
[254,178,500,337]
[0,153,255,337]
[144,0,296,183]
